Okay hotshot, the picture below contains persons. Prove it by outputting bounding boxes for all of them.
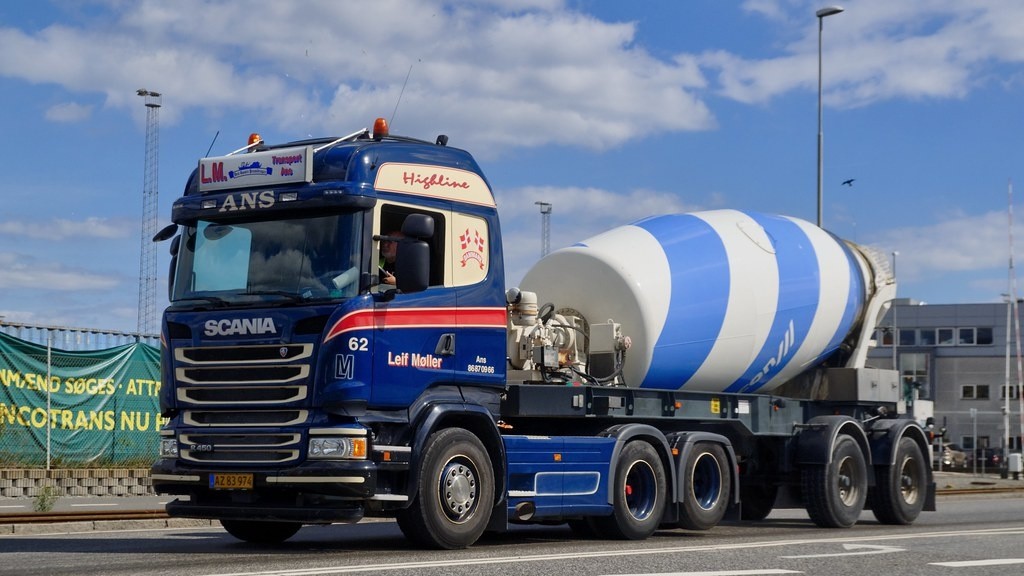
[349,231,404,286]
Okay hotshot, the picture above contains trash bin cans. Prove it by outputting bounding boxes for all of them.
[1009,454,1022,480]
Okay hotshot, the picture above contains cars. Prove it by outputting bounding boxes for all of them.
[934,441,969,471]
[966,446,1004,472]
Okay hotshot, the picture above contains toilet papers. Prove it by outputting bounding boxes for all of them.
[331,266,360,291]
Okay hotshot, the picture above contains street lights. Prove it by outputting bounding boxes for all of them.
[814,5,849,229]
[889,251,901,372]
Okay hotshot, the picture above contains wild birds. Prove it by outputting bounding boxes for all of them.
[841,179,856,187]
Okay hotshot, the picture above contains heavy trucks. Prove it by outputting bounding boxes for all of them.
[144,117,945,550]
